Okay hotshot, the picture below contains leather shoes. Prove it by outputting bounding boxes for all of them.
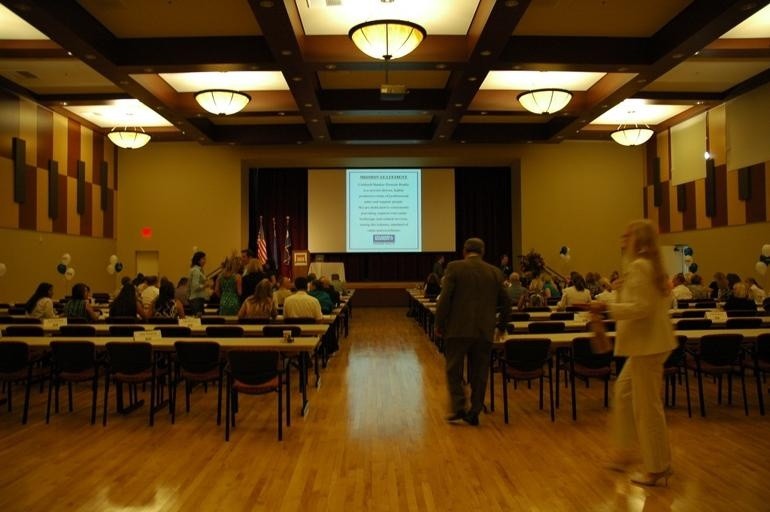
[449,409,478,425]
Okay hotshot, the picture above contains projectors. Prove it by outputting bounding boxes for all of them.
[380,85,405,100]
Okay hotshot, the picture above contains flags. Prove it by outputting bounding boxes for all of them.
[283,219,292,266]
[273,221,278,270]
[257,221,268,266]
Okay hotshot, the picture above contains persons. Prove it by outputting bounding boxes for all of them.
[64,249,348,322]
[674,271,770,314]
[25,282,65,320]
[423,255,446,301]
[590,218,674,487]
[499,256,619,312]
[434,237,512,426]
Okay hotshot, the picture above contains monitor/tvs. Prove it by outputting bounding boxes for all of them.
[315,255,324,262]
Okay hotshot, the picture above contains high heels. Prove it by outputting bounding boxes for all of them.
[631,468,672,489]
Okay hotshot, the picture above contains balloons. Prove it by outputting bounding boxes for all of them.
[0,262,7,277]
[57,253,75,281]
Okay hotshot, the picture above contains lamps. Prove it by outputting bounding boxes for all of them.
[107,125,152,151]
[193,88,251,118]
[610,110,654,147]
[516,88,573,116]
[347,0,427,62]
